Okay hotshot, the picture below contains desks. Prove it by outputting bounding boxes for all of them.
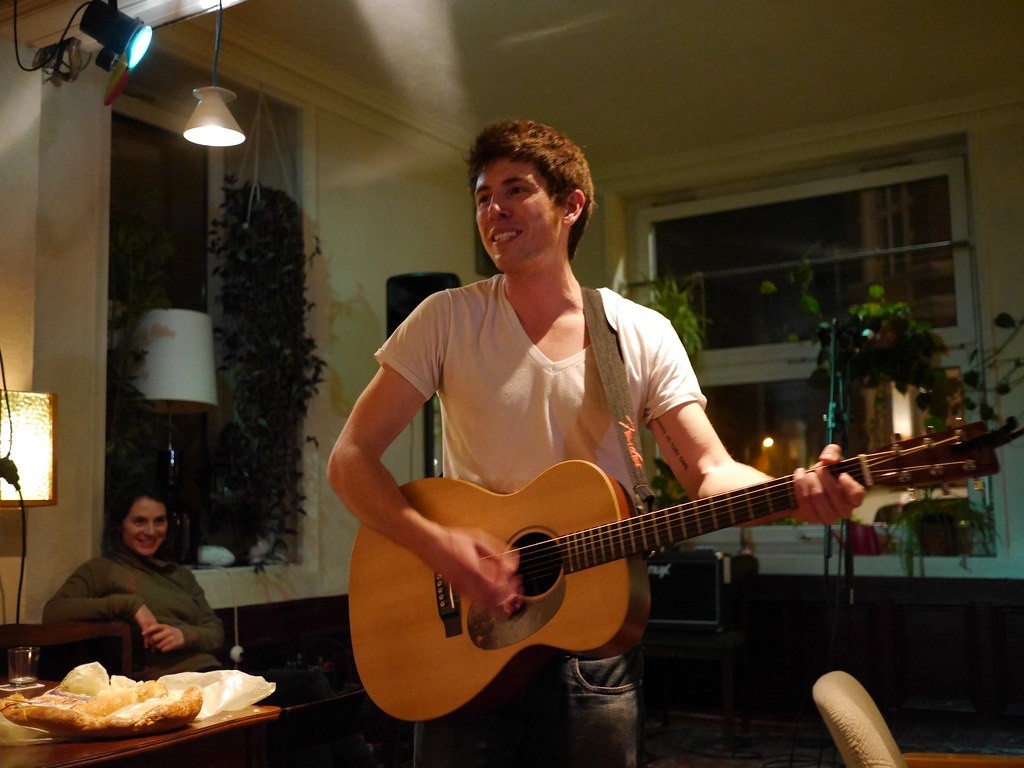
[0,679,281,768]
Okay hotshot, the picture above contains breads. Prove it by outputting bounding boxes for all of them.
[0,681,203,738]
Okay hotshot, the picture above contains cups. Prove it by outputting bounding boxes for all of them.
[6,645,40,688]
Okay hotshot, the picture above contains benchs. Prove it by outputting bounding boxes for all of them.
[644,628,757,752]
[0,595,396,768]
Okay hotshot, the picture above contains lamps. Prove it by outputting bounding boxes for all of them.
[182,0,246,147]
[385,271,460,479]
[121,307,217,567]
[0,390,57,509]
[39,1,153,72]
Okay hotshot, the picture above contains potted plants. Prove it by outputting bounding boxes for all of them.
[810,284,1023,434]
[885,487,1000,581]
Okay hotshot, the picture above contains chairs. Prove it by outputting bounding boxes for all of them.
[811,670,1024,768]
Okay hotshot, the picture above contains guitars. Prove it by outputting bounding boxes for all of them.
[342,406,1003,730]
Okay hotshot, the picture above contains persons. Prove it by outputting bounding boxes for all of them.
[44,483,379,768]
[328,118,865,768]
